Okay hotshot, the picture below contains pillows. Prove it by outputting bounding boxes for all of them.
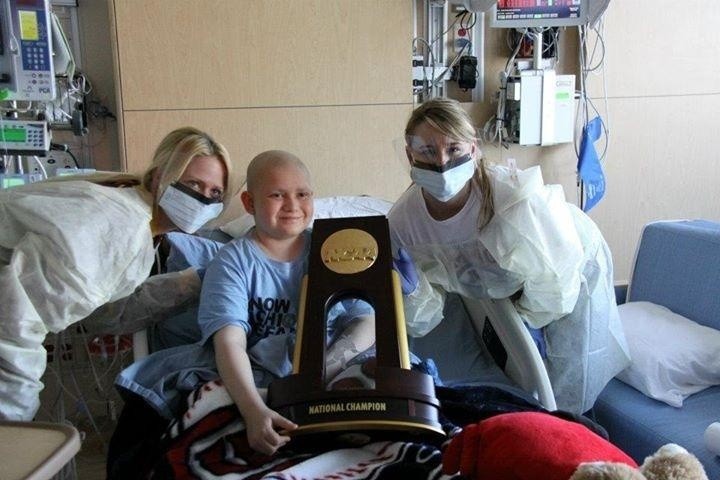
[612,301,719,408]
[220,194,394,238]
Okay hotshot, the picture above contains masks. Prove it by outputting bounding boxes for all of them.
[409,152,478,204]
[157,180,225,234]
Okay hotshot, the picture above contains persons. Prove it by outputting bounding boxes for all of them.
[385,97,632,414]
[1,125,209,422]
[197,149,374,457]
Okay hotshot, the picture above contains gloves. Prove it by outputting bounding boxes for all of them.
[393,247,419,297]
[524,321,548,360]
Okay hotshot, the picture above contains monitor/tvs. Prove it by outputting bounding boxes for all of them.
[491,0,587,29]
